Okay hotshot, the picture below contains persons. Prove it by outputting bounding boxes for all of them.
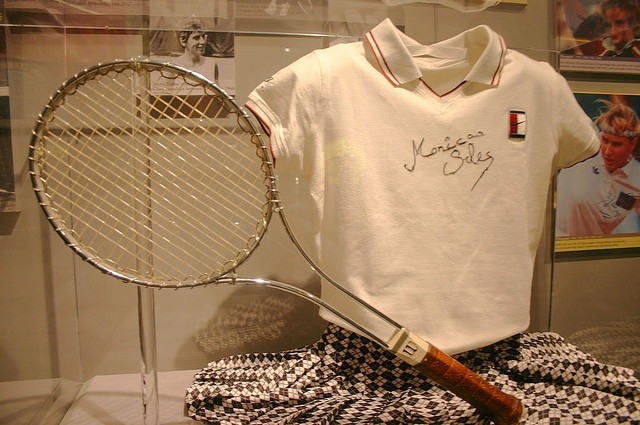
[578,1,639,59]
[556,102,640,237]
[157,19,221,92]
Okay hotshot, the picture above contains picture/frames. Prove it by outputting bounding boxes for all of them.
[144,1,236,99]
[552,82,639,252]
[555,1,640,75]
